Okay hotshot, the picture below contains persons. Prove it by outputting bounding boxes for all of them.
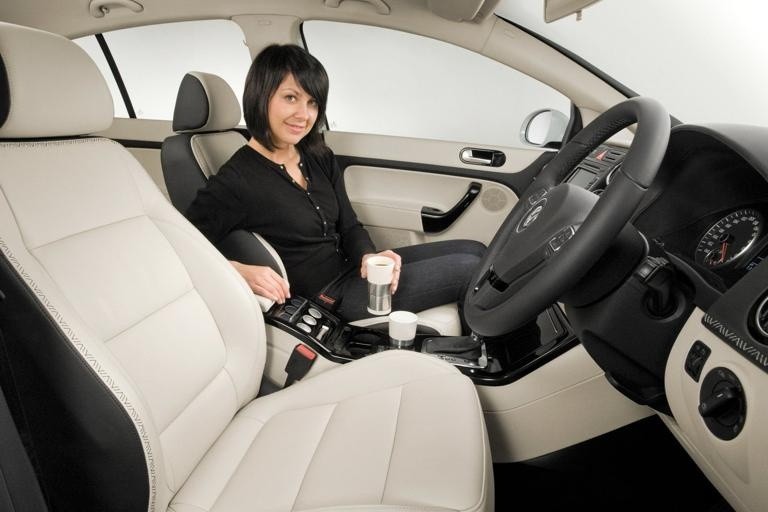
[185,43,541,369]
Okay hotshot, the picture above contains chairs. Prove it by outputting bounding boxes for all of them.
[156,63,466,344]
[3,21,492,510]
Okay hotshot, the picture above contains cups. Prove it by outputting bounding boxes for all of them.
[387,311,418,348]
[366,256,396,316]
